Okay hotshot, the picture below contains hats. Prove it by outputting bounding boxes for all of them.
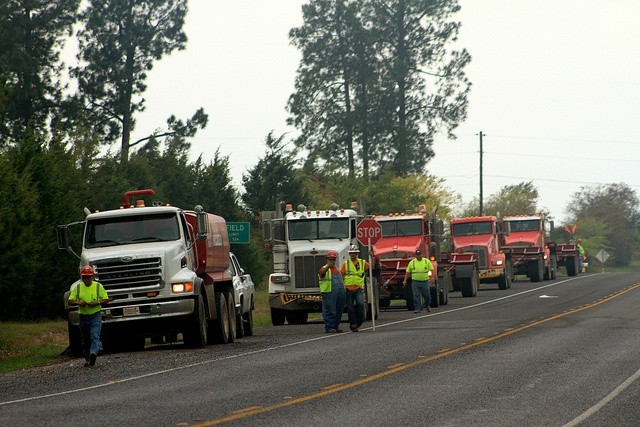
[415,250,422,254]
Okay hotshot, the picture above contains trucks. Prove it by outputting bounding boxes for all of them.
[440,215,515,289]
[268,198,380,325]
[365,203,452,308]
[56,189,239,348]
[501,213,581,281]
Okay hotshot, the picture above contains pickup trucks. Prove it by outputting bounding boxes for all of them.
[228,251,256,338]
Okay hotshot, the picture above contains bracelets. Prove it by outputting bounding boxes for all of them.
[97,301,101,306]
[74,301,78,305]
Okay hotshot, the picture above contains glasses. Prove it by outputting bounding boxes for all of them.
[329,258,336,260]
[83,276,92,278]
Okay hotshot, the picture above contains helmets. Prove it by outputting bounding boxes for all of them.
[347,244,360,252]
[327,250,337,257]
[81,265,94,275]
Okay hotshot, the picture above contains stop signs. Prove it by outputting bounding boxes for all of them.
[356,219,383,246]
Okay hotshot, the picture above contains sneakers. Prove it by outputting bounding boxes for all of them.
[326,328,335,333]
[414,308,420,312]
[89,351,97,365]
[335,326,344,332]
[427,305,431,311]
[350,324,358,331]
[84,359,90,366]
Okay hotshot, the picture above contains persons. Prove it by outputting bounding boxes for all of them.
[67,265,110,368]
[576,238,587,274]
[340,244,370,332]
[317,250,345,334]
[402,249,434,314]
